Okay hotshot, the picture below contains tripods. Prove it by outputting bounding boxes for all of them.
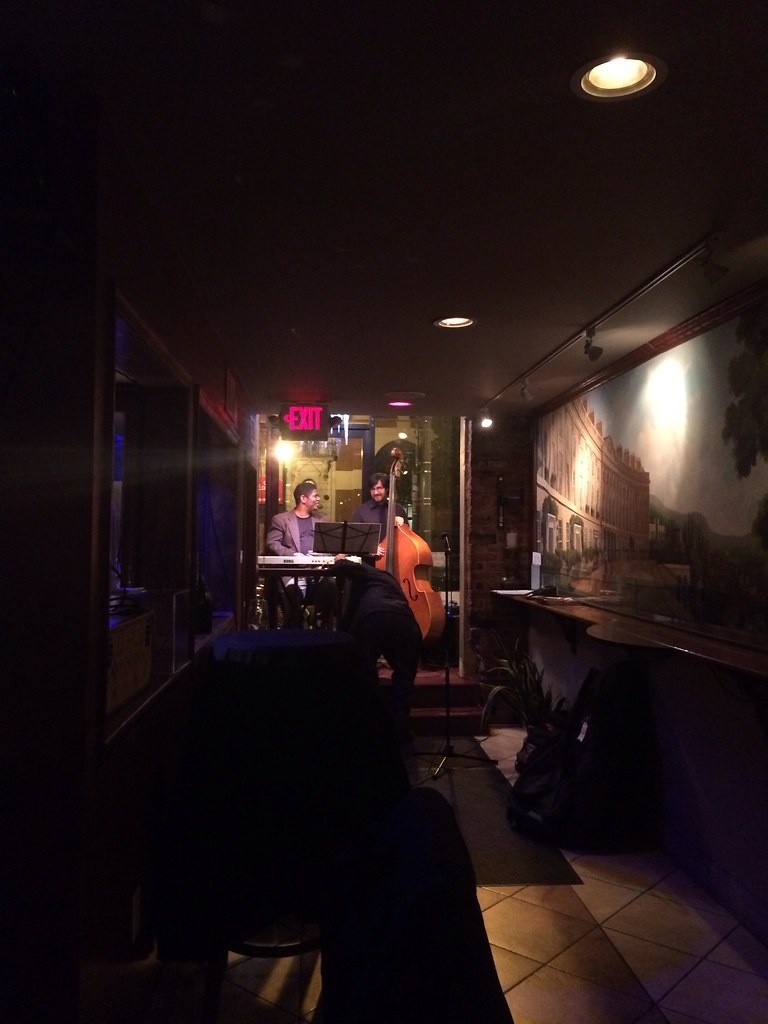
[417,550,499,777]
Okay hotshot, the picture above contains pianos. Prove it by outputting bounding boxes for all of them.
[258,556,362,568]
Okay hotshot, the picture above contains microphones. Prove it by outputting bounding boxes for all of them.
[440,532,451,552]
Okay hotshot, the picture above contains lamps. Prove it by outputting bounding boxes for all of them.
[697,250,728,285]
[479,405,493,427]
[583,328,602,362]
[521,378,535,402]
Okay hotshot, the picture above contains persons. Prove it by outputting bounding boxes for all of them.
[263,483,337,629]
[328,559,422,728]
[352,473,410,546]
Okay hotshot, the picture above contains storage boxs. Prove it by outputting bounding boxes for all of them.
[108,608,154,717]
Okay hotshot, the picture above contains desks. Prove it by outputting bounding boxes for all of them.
[258,565,331,627]
[215,628,355,662]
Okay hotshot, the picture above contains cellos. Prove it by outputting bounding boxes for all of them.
[374,446,445,644]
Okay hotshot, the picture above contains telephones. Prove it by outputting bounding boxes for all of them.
[496,477,524,506]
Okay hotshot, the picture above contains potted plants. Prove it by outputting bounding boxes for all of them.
[482,624,568,773]
